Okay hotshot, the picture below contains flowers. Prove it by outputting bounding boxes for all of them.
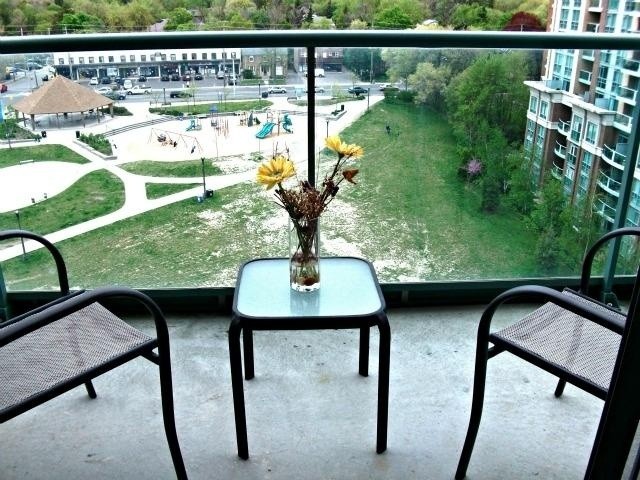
[256,136,365,263]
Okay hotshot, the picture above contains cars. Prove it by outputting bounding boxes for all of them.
[380,84,399,92]
[41,74,49,82]
[305,86,326,93]
[139,76,145,82]
[267,87,287,94]
[89,76,133,100]
[161,71,203,82]
[0,83,8,93]
[349,86,368,94]
[217,71,238,83]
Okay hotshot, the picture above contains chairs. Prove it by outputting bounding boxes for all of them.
[454,227,640,480]
[0,230,189,480]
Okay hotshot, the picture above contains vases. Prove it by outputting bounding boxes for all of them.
[289,208,321,293]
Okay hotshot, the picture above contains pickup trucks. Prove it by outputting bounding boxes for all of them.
[128,85,152,95]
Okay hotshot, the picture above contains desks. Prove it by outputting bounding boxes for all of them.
[226,259,391,460]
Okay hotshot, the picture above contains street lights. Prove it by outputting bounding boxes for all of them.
[230,51,237,95]
[258,82,261,98]
[324,118,330,139]
[199,156,207,194]
[14,210,28,255]
[366,88,370,111]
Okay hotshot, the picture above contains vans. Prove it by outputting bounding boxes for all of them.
[27,62,42,69]
[304,69,325,77]
